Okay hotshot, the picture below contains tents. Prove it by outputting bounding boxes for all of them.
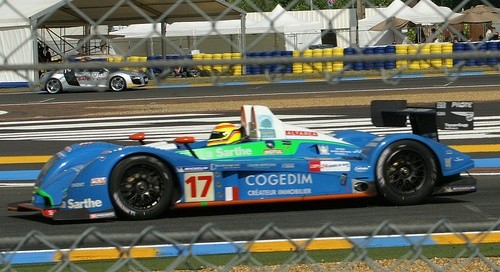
[110,5,321,57]
[368,0,500,31]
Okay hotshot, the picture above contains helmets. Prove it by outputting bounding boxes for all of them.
[205,123,241,146]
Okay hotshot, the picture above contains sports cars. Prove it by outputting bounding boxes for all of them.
[6,97,479,227]
[39,58,149,95]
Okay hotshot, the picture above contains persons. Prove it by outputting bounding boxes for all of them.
[401,27,500,44]
[45,46,51,62]
[206,124,241,148]
